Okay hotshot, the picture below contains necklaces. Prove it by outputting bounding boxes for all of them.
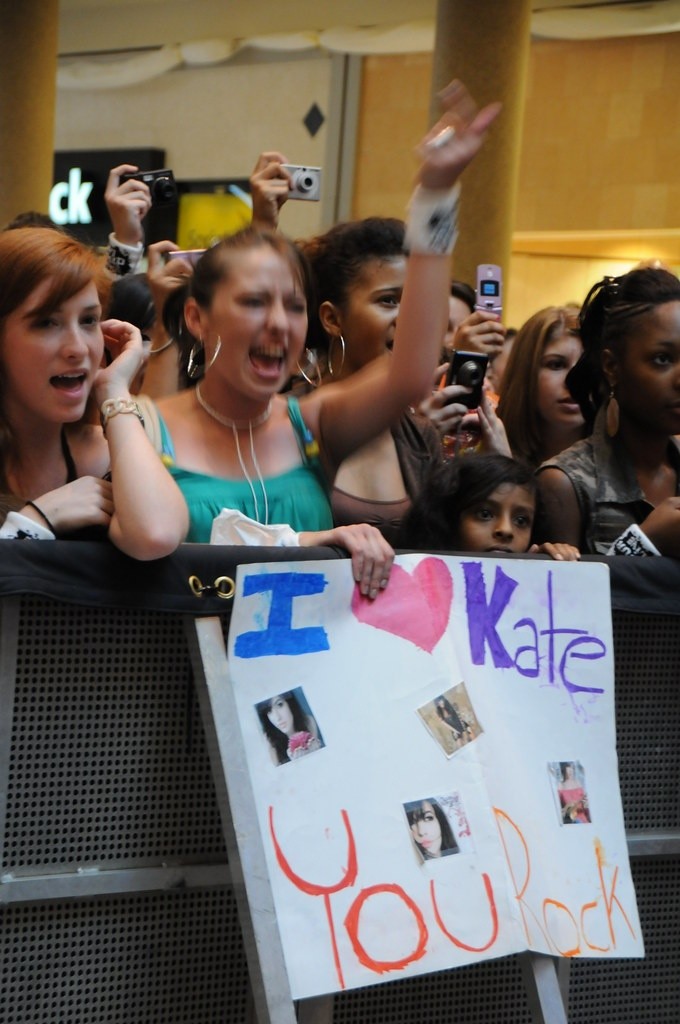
[192,381,272,429]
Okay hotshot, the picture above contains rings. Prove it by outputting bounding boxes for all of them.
[428,126,454,149]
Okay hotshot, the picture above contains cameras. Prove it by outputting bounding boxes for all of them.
[278,164,324,201]
[119,169,177,210]
[442,350,488,410]
[164,248,206,270]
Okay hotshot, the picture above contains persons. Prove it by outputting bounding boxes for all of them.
[403,798,460,860]
[0,77,680,603]
[433,695,471,749]
[548,761,591,824]
[254,690,318,767]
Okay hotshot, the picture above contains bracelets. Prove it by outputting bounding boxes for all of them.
[102,231,144,284]
[99,398,144,439]
[24,501,58,536]
[399,180,461,255]
[605,522,663,557]
[148,326,182,353]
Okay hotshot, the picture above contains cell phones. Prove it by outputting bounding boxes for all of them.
[472,263,504,317]
